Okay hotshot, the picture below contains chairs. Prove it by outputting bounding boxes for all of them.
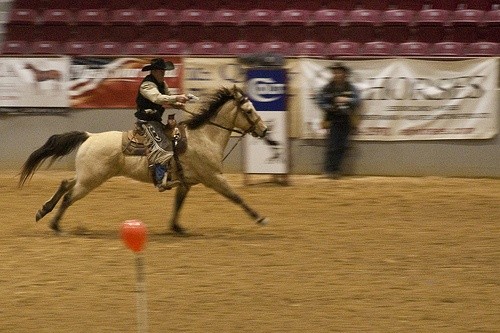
[1,0,500,56]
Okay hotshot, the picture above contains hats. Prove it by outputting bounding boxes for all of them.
[141,57,174,74]
[326,62,350,72]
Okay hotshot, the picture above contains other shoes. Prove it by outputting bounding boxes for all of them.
[157,179,184,192]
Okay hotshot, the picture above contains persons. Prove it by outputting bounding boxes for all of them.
[314,61,359,179]
[133,58,190,192]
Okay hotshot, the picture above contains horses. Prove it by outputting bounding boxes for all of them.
[23,63,61,84]
[15,84,271,236]
